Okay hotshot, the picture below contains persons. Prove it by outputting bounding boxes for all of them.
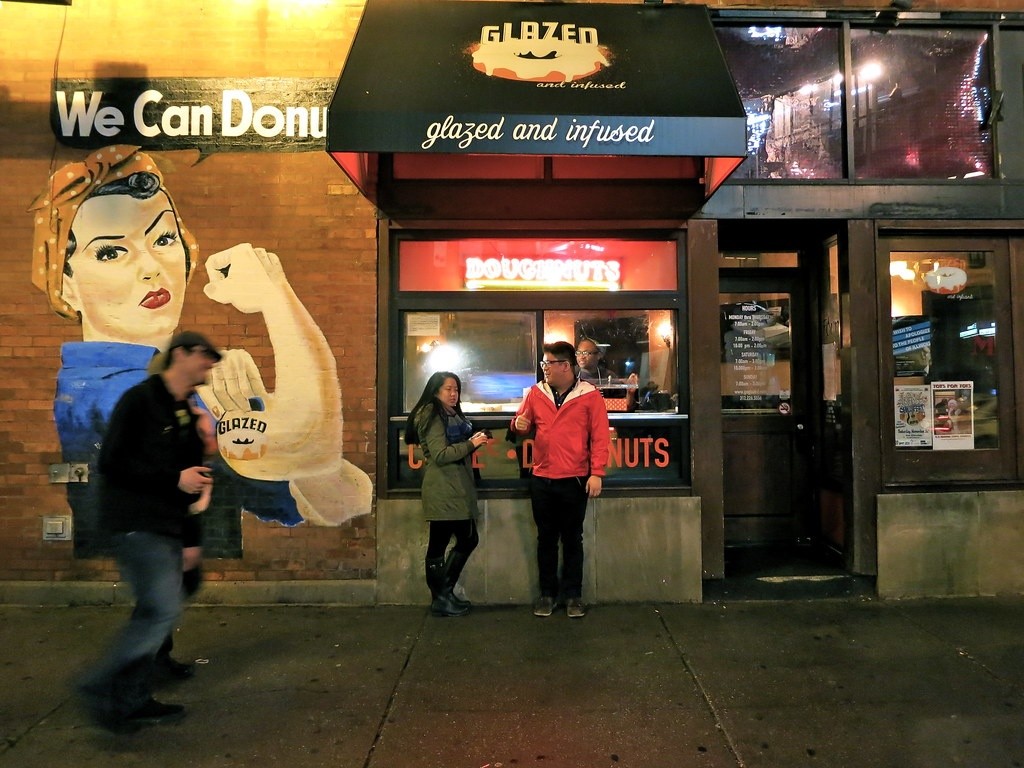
[82,329,223,732]
[404,370,488,617]
[575,336,620,384]
[509,342,610,618]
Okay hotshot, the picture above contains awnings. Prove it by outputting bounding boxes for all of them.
[323,0,748,209]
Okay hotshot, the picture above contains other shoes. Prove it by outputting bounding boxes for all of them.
[534,596,557,616]
[157,659,196,685]
[566,598,585,617]
[131,704,185,724]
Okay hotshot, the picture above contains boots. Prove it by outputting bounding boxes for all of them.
[424,555,472,608]
[430,551,469,618]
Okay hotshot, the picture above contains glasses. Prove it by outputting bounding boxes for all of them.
[575,350,599,357]
[539,360,572,370]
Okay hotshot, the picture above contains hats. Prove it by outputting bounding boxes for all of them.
[168,331,222,363]
